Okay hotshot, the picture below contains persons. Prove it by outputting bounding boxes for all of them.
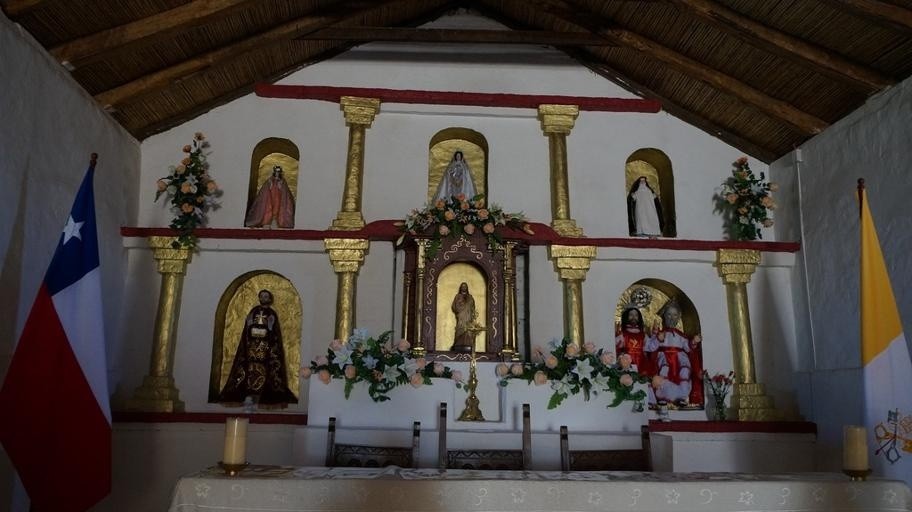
[431,151,478,203]
[627,176,664,236]
[218,290,298,404]
[451,282,476,345]
[246,166,294,228]
[615,309,657,406]
[652,304,702,410]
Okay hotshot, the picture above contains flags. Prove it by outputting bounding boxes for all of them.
[858,189,912,479]
[0,164,113,512]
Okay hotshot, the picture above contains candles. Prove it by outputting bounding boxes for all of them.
[221,414,252,465]
[841,423,869,471]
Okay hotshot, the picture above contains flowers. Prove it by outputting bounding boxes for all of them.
[293,325,472,406]
[151,130,228,254]
[698,364,739,420]
[489,334,665,417]
[714,154,781,248]
[389,189,537,263]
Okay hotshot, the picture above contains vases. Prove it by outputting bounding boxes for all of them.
[708,396,730,422]
